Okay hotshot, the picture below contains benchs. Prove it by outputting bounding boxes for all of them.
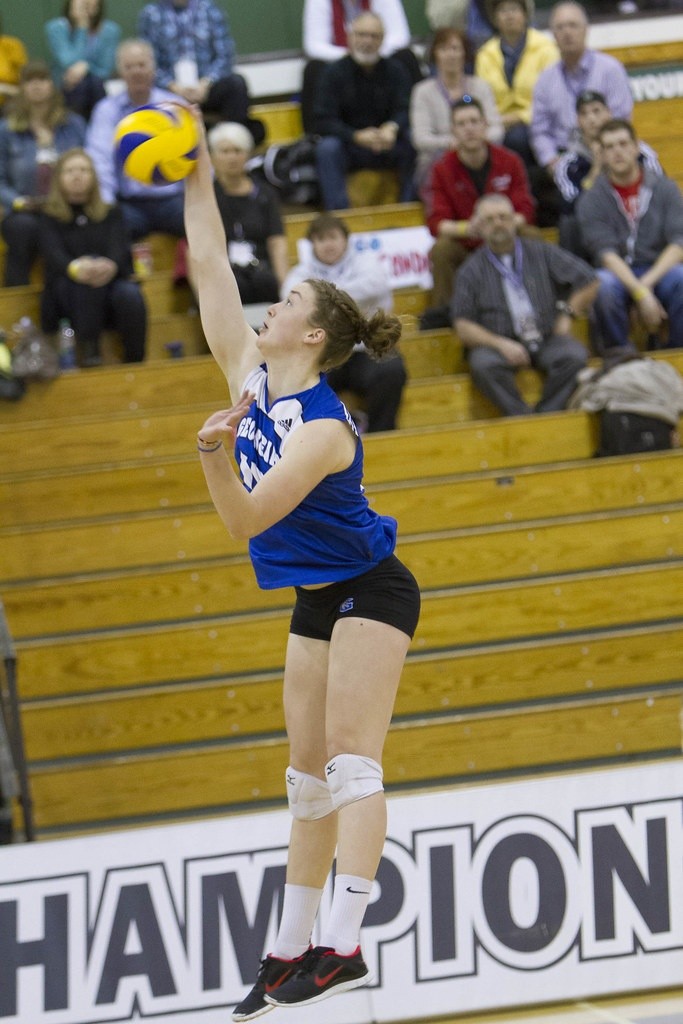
[0,41,683,834]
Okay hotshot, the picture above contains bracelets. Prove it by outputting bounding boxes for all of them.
[555,300,577,319]
[582,181,591,190]
[10,199,25,212]
[198,437,218,444]
[632,286,648,301]
[458,221,468,238]
[67,261,80,283]
[198,442,222,452]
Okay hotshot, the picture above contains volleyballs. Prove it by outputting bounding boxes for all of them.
[113,103,199,188]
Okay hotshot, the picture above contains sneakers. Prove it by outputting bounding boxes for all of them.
[232,944,313,1022]
[263,946,373,1007]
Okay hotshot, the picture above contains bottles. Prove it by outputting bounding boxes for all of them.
[7,316,77,379]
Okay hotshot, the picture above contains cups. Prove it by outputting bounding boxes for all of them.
[130,242,154,279]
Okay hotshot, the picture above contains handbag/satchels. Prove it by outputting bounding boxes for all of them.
[247,141,316,205]
[600,409,670,454]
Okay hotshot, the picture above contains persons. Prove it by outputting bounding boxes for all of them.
[420,98,537,329]
[529,0,635,220]
[137,1,252,115]
[209,120,285,304]
[184,102,423,1022]
[573,118,683,355]
[474,0,559,166]
[81,38,192,239]
[553,89,663,247]
[449,194,600,413]
[312,9,417,211]
[39,148,145,366]
[302,0,422,134]
[277,215,409,433]
[41,0,122,116]
[0,34,85,287]
[408,31,506,188]
